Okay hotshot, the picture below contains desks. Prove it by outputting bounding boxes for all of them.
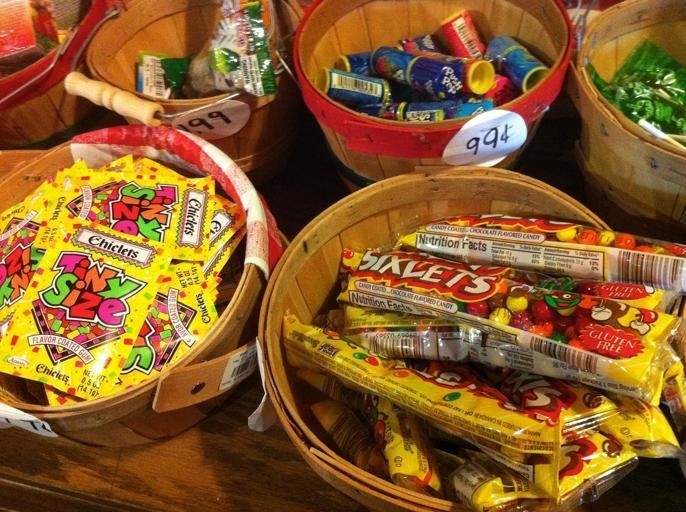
[1,133,686,511]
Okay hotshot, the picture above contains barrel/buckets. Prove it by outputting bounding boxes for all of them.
[1,0,118,150]
[292,1,575,192]
[0,124,289,448]
[64,1,300,185]
[258,165,618,512]
[570,0,685,224]
